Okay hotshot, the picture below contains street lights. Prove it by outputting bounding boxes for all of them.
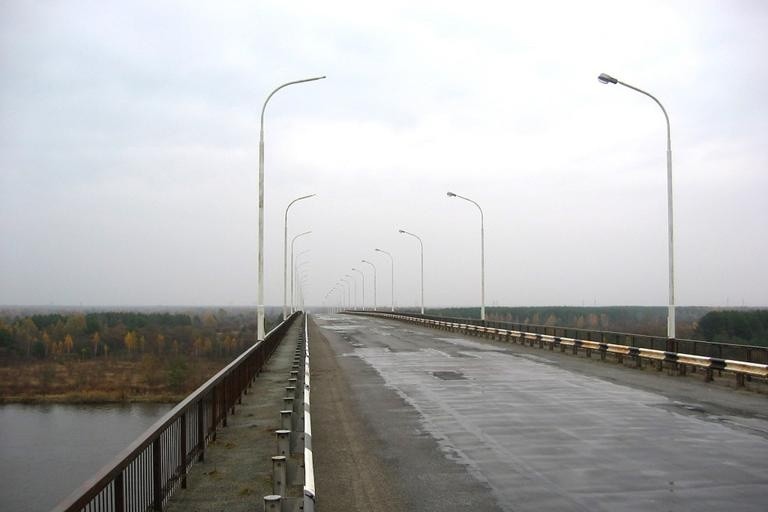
[447,192,485,320]
[258,75,327,341]
[399,230,424,314]
[598,73,675,339]
[352,268,364,311]
[361,260,376,311]
[325,268,356,312]
[375,249,394,311]
